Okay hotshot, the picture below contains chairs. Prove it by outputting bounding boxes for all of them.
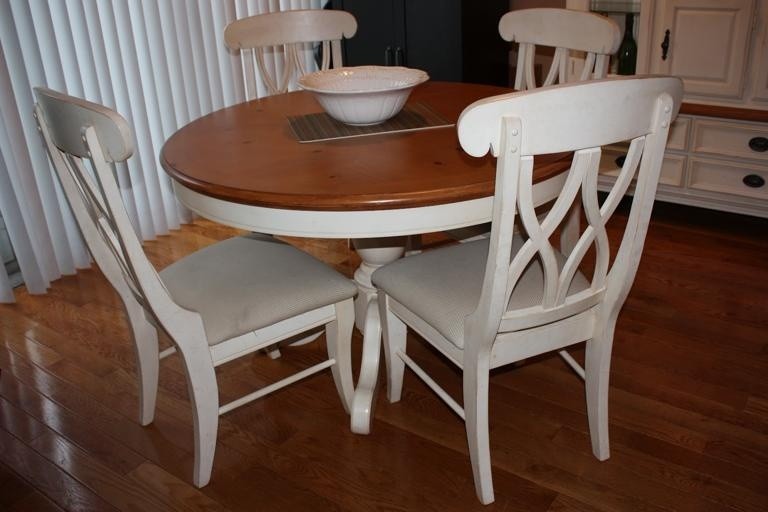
[373,76,684,505]
[410,8,621,267]
[224,10,359,104]
[31,86,357,487]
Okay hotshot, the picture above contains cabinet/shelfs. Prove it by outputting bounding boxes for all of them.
[564,0,767,110]
[332,0,509,87]
[596,104,768,222]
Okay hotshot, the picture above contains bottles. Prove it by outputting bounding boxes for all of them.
[617,13,637,75]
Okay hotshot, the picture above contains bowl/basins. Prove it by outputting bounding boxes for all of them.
[295,66,430,127]
